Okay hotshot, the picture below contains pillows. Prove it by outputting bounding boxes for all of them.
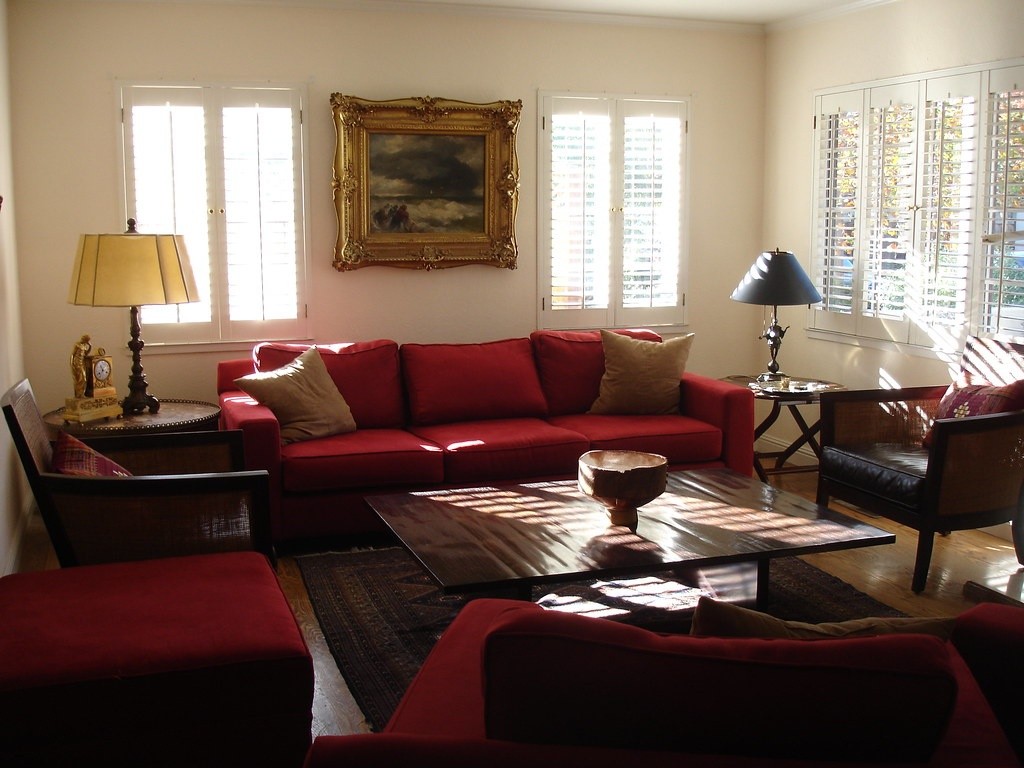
[400,337,550,430]
[232,343,356,447]
[480,605,960,764]
[48,430,135,477]
[921,379,1024,450]
[688,596,956,643]
[253,338,408,429]
[530,329,663,419]
[587,328,696,416]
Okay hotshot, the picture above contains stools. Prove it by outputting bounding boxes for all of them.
[0,552,314,768]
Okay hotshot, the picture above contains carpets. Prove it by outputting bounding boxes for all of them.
[290,546,914,733]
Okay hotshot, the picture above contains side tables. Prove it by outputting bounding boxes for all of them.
[42,398,222,438]
[719,375,848,485]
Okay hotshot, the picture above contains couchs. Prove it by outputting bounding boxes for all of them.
[216,358,755,543]
[952,601,1024,768]
[303,598,1024,768]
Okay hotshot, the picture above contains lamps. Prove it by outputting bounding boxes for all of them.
[65,218,202,417]
[729,248,823,382]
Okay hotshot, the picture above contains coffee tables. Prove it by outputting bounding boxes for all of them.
[364,466,896,612]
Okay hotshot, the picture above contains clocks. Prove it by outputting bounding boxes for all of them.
[62,333,123,427]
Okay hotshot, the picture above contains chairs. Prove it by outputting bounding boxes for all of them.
[816,334,1024,594]
[0,377,277,572]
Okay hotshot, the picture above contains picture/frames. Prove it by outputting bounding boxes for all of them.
[329,91,524,272]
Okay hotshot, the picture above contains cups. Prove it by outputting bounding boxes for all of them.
[780,375,791,389]
